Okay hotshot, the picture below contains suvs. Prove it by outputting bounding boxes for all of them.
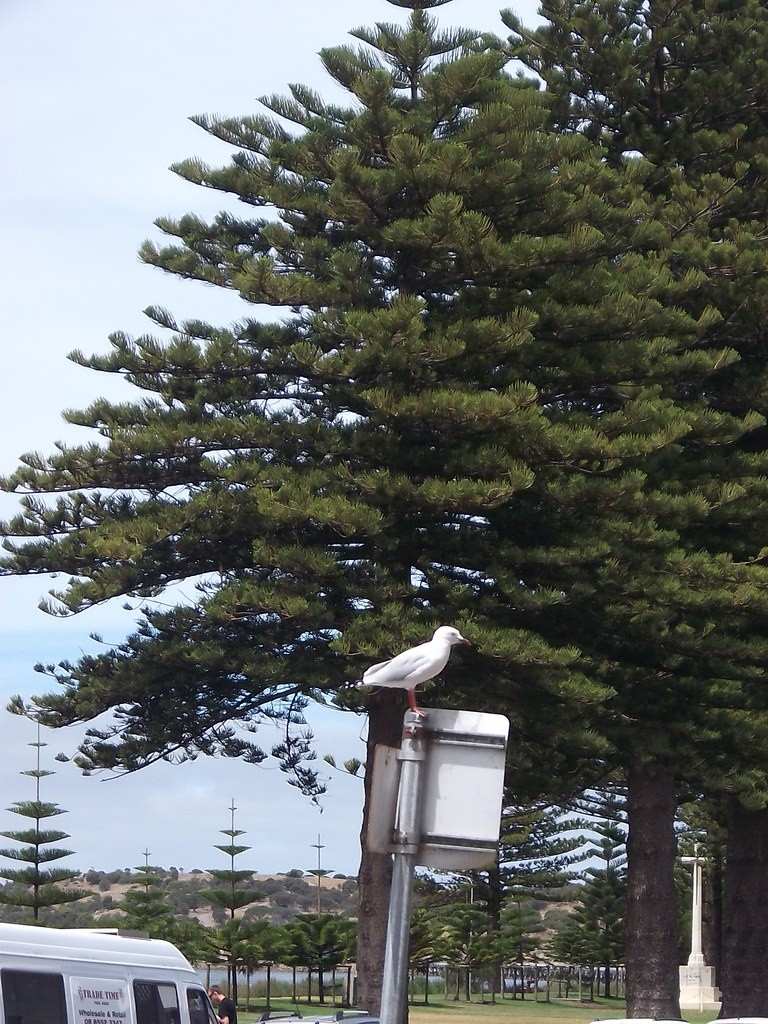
[253,1010,380,1024]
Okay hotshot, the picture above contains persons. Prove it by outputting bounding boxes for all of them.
[207,985,237,1024]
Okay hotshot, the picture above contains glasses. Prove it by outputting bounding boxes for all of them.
[209,993,214,998]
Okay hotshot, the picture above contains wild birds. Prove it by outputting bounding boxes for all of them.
[356,624,476,726]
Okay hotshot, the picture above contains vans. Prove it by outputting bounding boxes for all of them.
[0,923,219,1024]
